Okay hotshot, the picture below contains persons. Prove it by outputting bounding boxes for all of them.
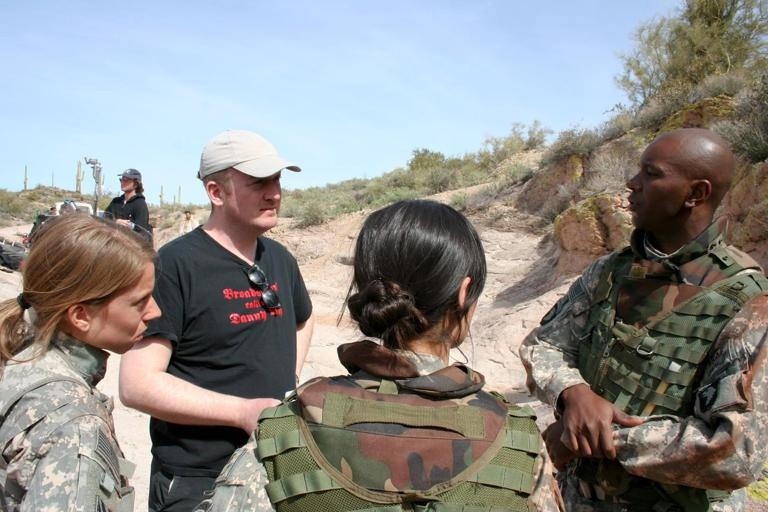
[183,211,198,235]
[0,213,161,512]
[520,129,767,511]
[105,168,149,238]
[208,199,566,509]
[119,129,315,510]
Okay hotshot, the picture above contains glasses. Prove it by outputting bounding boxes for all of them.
[241,263,283,310]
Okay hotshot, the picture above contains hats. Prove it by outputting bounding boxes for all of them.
[117,168,142,181]
[198,128,302,180]
